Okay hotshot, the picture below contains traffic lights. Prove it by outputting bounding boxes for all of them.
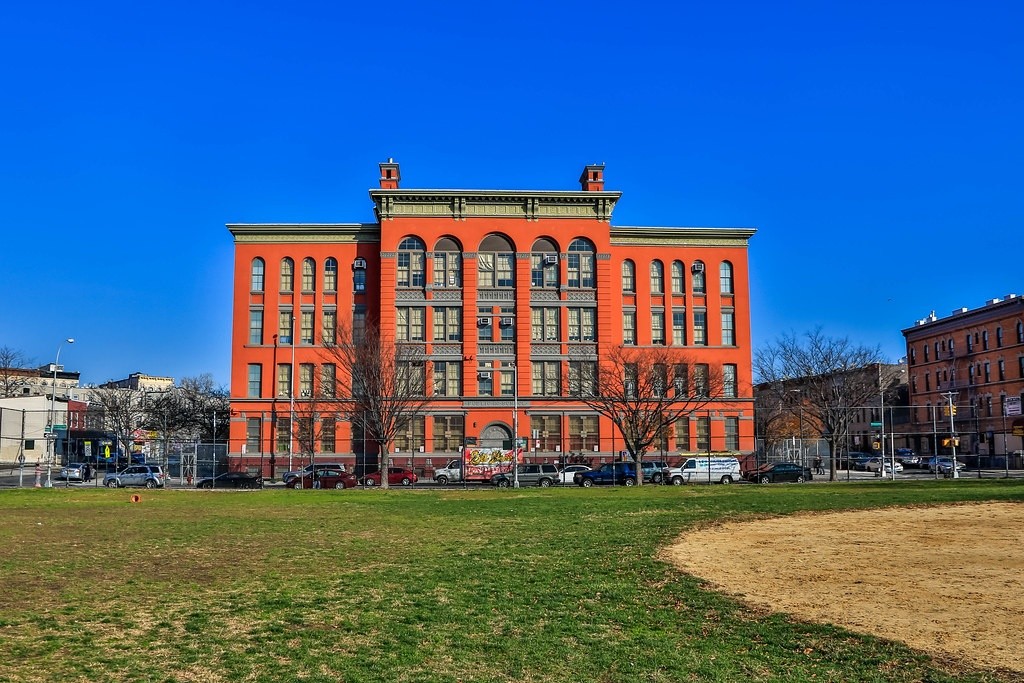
[944,406,950,416]
[952,405,957,416]
[951,439,959,447]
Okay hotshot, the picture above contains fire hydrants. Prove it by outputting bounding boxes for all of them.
[34,462,43,488]
[187,475,192,485]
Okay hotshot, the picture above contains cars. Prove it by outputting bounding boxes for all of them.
[556,464,593,484]
[60,462,97,481]
[360,466,418,486]
[744,460,814,484]
[837,447,967,475]
[287,468,358,490]
[196,470,262,490]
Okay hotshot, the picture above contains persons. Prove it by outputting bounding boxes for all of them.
[84,463,91,482]
[814,456,826,475]
[187,475,192,486]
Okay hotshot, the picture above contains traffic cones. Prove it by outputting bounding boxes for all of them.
[130,494,143,503]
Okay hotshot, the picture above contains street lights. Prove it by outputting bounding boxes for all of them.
[790,389,805,483]
[46,338,75,487]
[880,369,905,478]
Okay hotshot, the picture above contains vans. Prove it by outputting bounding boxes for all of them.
[432,459,463,486]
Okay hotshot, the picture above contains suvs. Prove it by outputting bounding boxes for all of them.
[282,462,346,482]
[102,464,164,489]
[640,460,668,484]
[489,462,561,488]
[573,461,637,487]
[662,456,743,486]
